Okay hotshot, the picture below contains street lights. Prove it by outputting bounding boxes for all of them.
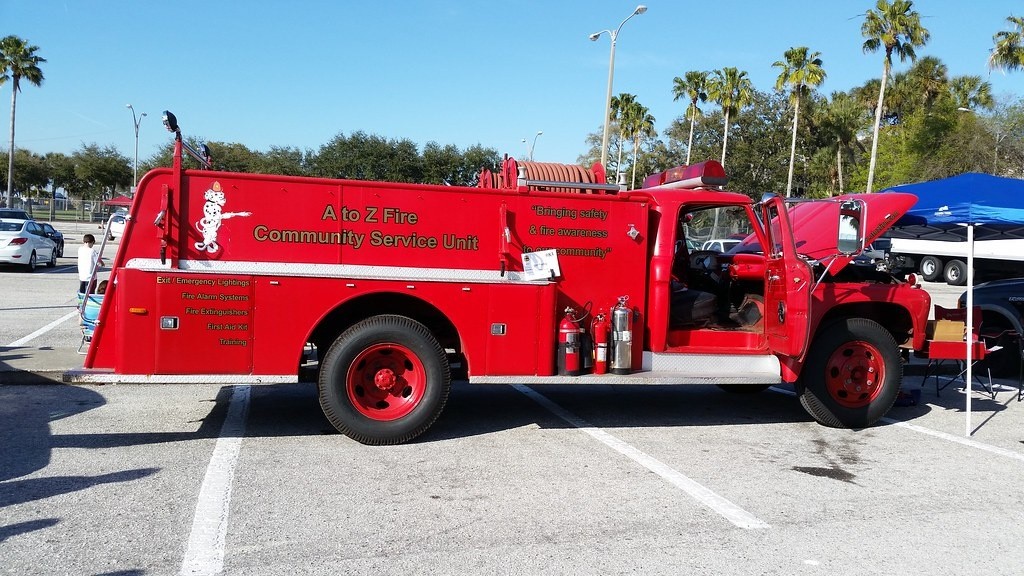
[127,104,146,195]
[589,5,648,174]
[521,131,543,161]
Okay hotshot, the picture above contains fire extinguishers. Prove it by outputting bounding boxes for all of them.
[591,313,610,375]
[558,304,580,370]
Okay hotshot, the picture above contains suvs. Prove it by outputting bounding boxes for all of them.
[703,239,742,254]
[0,208,33,220]
[107,212,128,240]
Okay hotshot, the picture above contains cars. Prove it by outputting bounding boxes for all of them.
[85,204,91,210]
[37,224,62,258]
[957,278,1024,376]
[0,218,58,271]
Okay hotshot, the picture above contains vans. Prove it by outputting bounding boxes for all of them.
[861,238,891,273]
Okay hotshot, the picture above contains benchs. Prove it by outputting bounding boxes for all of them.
[77,286,106,354]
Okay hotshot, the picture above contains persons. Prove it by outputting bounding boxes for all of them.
[77,234,105,294]
[85,279,108,343]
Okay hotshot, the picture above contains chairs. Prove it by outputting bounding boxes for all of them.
[669,289,718,330]
[914,305,1024,399]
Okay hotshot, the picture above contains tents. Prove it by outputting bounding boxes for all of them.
[878,172,1024,437]
[101,196,133,207]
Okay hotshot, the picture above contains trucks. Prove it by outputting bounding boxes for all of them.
[893,216,1024,286]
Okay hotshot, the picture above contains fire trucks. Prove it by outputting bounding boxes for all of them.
[61,110,930,446]
